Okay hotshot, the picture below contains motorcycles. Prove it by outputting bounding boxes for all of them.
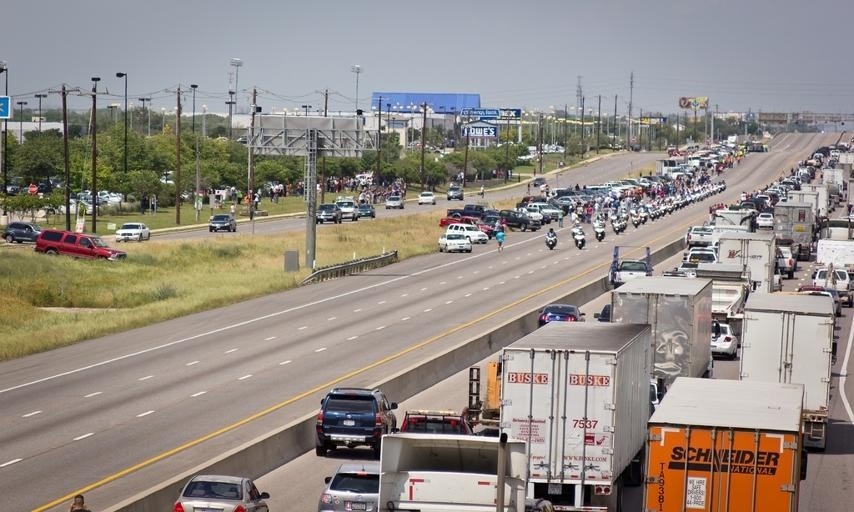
[575,231,585,250]
[545,234,556,250]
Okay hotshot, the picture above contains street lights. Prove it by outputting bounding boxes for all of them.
[0,68,7,217]
[158,106,166,135]
[242,103,262,212]
[90,76,98,232]
[173,105,178,132]
[229,56,243,113]
[523,95,714,171]
[115,72,128,175]
[200,104,208,135]
[16,100,28,146]
[301,105,312,115]
[225,100,236,140]
[350,62,360,113]
[188,83,198,135]
[374,98,512,188]
[107,104,116,119]
[33,94,47,132]
[137,96,151,134]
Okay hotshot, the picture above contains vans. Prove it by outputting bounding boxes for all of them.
[334,199,358,221]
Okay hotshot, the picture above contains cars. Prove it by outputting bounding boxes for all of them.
[710,322,738,358]
[537,304,585,327]
[161,170,233,203]
[28,228,126,264]
[115,223,151,243]
[592,304,610,322]
[514,134,770,240]
[208,214,237,233]
[7,176,140,215]
[664,133,854,323]
[515,207,542,221]
[173,474,272,512]
[417,192,436,205]
[359,205,375,218]
[385,195,405,209]
[315,203,341,224]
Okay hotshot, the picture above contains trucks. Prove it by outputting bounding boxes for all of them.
[468,362,499,423]
[644,376,810,510]
[497,323,667,511]
[739,292,835,454]
[611,277,713,407]
[377,429,530,512]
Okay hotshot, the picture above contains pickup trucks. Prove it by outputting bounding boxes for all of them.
[399,408,472,436]
[440,217,493,240]
[615,259,652,288]
[446,204,499,222]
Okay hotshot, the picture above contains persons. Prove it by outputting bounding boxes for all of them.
[480,185,484,199]
[457,170,463,184]
[138,192,148,215]
[191,170,409,218]
[148,192,158,215]
[526,180,531,195]
[495,229,504,253]
[68,494,88,512]
[540,140,815,270]
[493,218,507,238]
[492,166,520,183]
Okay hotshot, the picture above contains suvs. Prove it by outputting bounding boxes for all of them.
[446,187,464,201]
[446,223,488,245]
[315,462,380,511]
[315,387,398,455]
[2,222,40,244]
[437,232,472,254]
[499,209,540,233]
